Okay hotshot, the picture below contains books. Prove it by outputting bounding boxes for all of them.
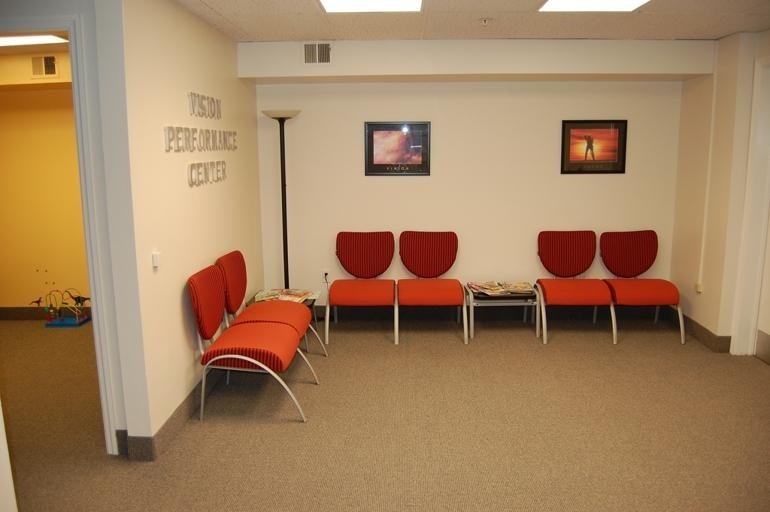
[467,281,535,297]
[254,288,321,304]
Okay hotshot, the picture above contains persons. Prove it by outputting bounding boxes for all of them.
[584,134,595,160]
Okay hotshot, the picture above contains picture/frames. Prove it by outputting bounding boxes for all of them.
[560,120,627,174]
[363,122,431,176]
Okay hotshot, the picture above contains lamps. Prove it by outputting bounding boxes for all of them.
[263,109,302,292]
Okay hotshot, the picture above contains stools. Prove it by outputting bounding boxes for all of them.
[463,283,541,339]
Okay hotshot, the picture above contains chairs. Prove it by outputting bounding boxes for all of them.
[186,249,327,423]
[531,230,688,346]
[325,231,470,345]
